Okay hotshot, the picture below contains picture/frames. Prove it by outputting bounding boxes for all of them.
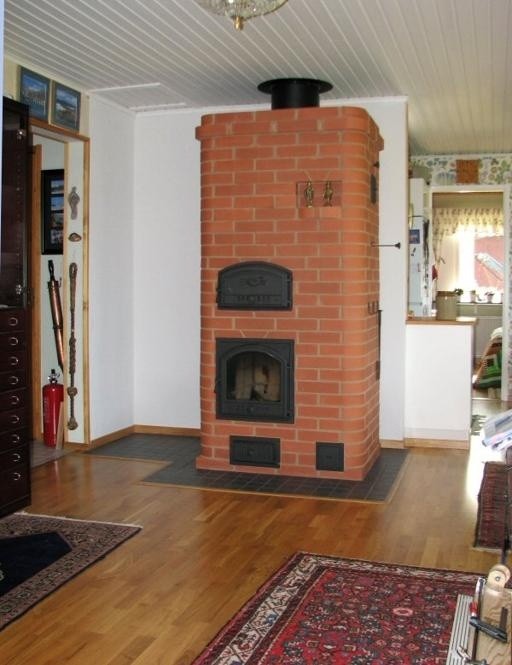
[471,461,512,553]
[40,168,64,255]
[14,64,51,123]
[51,80,81,133]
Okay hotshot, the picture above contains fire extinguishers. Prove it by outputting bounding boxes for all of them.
[42,369,64,448]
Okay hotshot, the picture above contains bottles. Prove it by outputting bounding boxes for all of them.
[436,291,457,320]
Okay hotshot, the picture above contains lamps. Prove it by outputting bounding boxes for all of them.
[192,0,288,30]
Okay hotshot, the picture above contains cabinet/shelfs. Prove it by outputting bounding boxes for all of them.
[0,95,32,519]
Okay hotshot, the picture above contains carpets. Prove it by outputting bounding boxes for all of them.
[189,550,512,665]
[0,509,143,631]
